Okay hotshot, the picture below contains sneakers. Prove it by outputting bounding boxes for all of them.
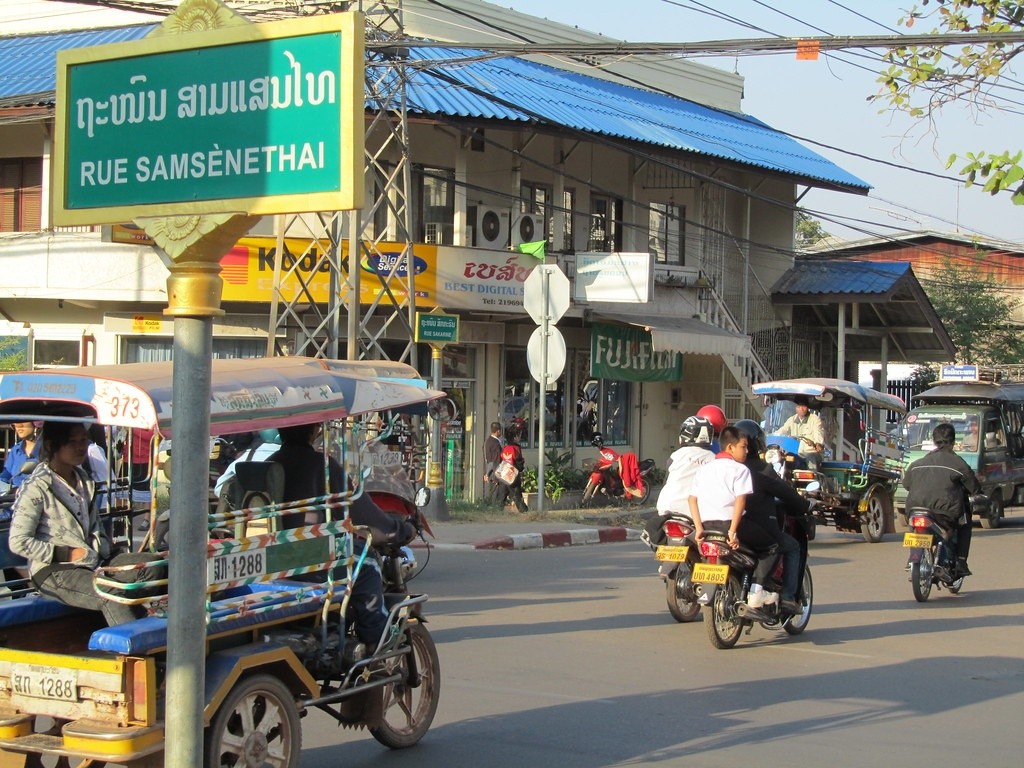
[747,589,779,609]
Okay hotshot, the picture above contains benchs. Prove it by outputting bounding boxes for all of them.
[86,528,318,655]
[214,578,346,605]
[0,516,112,628]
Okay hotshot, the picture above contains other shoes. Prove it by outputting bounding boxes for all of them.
[780,598,803,615]
[956,560,971,575]
[365,633,409,658]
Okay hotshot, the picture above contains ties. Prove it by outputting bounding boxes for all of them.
[714,451,735,460]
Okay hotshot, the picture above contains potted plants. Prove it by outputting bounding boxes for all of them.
[510,444,609,511]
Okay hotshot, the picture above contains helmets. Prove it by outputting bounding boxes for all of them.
[258,429,283,445]
[678,416,714,450]
[696,405,728,434]
[733,418,768,454]
[591,432,604,448]
[9,420,46,431]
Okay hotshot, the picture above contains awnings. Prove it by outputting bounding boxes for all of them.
[596,308,753,358]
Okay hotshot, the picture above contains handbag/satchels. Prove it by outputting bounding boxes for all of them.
[494,460,519,487]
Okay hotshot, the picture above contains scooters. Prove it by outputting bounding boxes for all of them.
[576,432,658,509]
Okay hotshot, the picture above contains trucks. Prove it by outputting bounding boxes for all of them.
[883,381,1023,528]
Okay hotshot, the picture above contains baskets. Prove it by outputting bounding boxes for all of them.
[582,457,603,472]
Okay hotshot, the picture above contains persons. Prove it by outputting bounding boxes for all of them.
[959,418,988,451]
[485,421,529,512]
[213,429,283,499]
[900,424,985,582]
[768,395,826,469]
[214,429,256,477]
[0,400,169,626]
[261,422,417,654]
[653,403,812,617]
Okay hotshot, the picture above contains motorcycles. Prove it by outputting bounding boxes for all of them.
[652,512,708,624]
[749,380,915,540]
[694,480,824,650]
[0,357,451,767]
[902,503,969,601]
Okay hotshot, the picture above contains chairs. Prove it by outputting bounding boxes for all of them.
[986,432,997,449]
[232,461,286,532]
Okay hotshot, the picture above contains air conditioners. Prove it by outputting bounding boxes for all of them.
[425,223,472,247]
[466,205,510,250]
[511,211,544,250]
